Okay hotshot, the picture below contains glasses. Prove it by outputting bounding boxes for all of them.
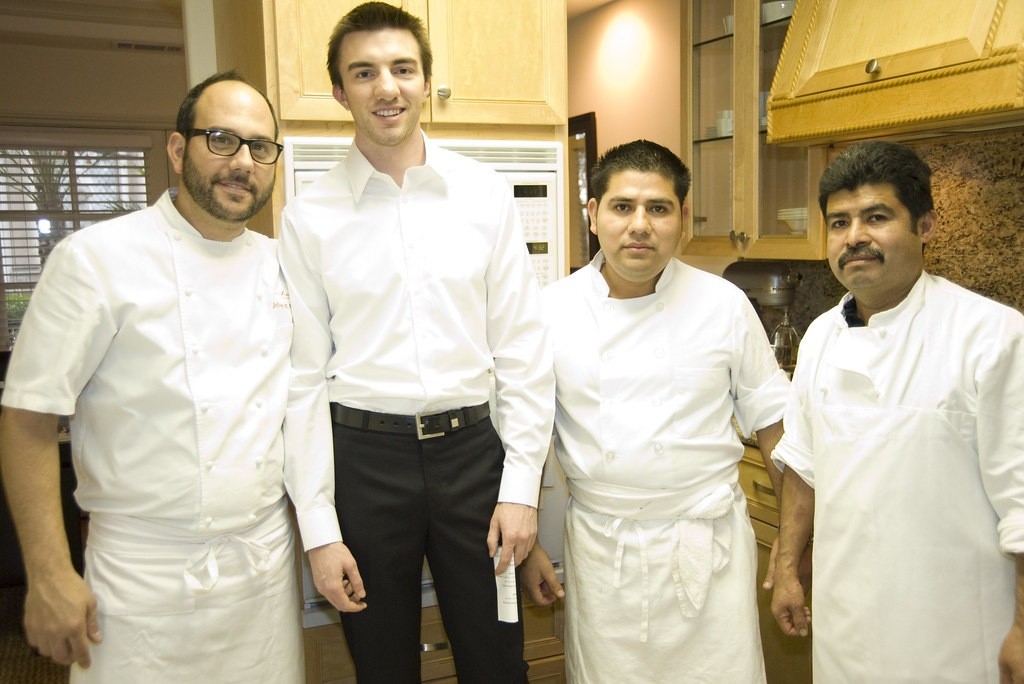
[183,128,284,165]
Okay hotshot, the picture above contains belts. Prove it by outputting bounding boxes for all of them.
[330,401,490,441]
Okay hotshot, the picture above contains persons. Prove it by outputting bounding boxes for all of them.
[767,138,1023,684]
[281,3,555,684]
[1,68,303,684]
[510,140,793,684]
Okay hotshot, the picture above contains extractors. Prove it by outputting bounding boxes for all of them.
[766,0,1023,146]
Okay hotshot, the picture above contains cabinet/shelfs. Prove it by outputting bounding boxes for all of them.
[680,0,827,260]
[738,443,813,684]
[272,1,566,126]
[767,0,1024,148]
[301,584,567,684]
